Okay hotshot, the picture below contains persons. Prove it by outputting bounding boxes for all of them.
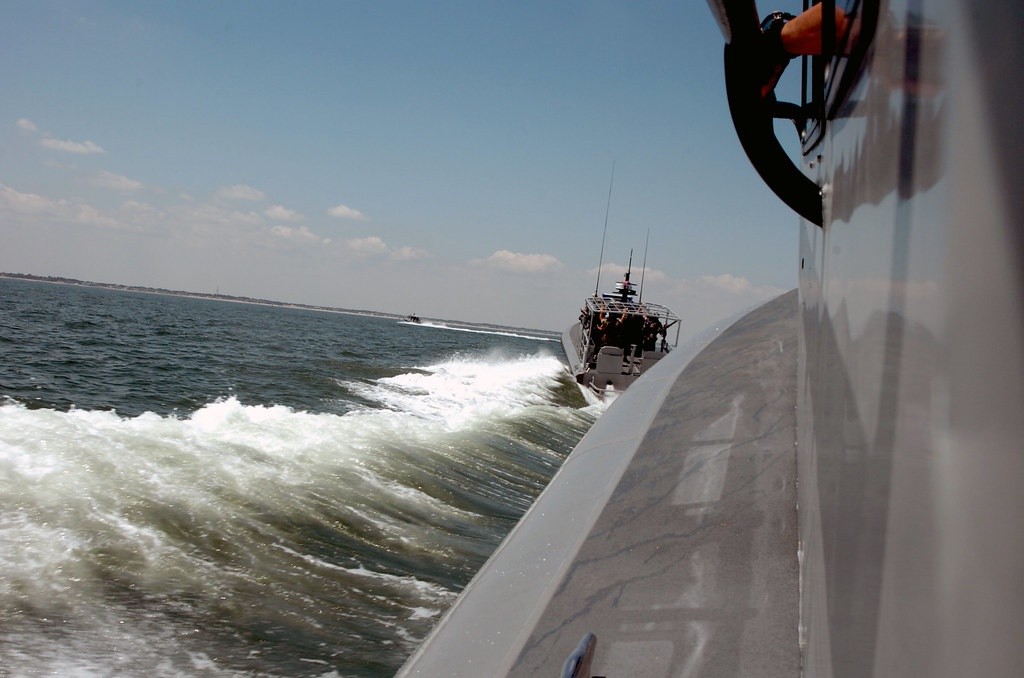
[580,304,667,362]
[760,0,862,98]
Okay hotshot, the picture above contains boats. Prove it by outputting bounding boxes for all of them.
[406,314,422,324]
[559,176,683,407]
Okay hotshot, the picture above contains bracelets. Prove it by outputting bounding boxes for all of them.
[761,11,802,60]
